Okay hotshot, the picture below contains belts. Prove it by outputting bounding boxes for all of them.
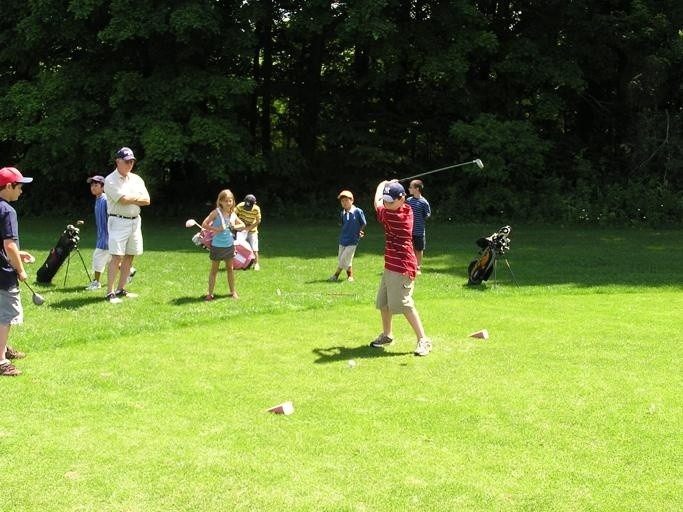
[108,214,138,219]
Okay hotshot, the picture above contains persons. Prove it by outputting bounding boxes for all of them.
[103,147,151,303]
[403,179,431,275]
[83,175,135,291]
[0,167,44,377]
[182,188,247,300]
[326,191,368,280]
[232,194,263,270]
[368,178,432,356]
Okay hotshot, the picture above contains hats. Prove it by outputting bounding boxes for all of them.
[87,176,104,184]
[337,190,353,200]
[116,146,137,161]
[244,194,256,210]
[382,183,406,202]
[0,167,33,186]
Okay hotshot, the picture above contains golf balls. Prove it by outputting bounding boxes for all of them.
[348,360,355,367]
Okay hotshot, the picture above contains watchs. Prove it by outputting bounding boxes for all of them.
[134,197,137,202]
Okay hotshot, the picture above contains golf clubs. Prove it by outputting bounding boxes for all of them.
[66,220,84,245]
[397,159,484,180]
[496,226,510,238]
[1,252,45,305]
[185,219,203,230]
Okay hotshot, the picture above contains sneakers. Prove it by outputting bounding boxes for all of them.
[5,346,24,359]
[415,337,433,355]
[347,276,353,281]
[371,333,394,348]
[115,287,138,297]
[254,263,259,270]
[127,267,136,283]
[86,279,101,290]
[417,265,422,274]
[232,290,237,298]
[327,275,338,281]
[105,292,121,303]
[0,361,22,375]
[205,294,213,301]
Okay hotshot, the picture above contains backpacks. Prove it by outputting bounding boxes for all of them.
[199,208,225,249]
